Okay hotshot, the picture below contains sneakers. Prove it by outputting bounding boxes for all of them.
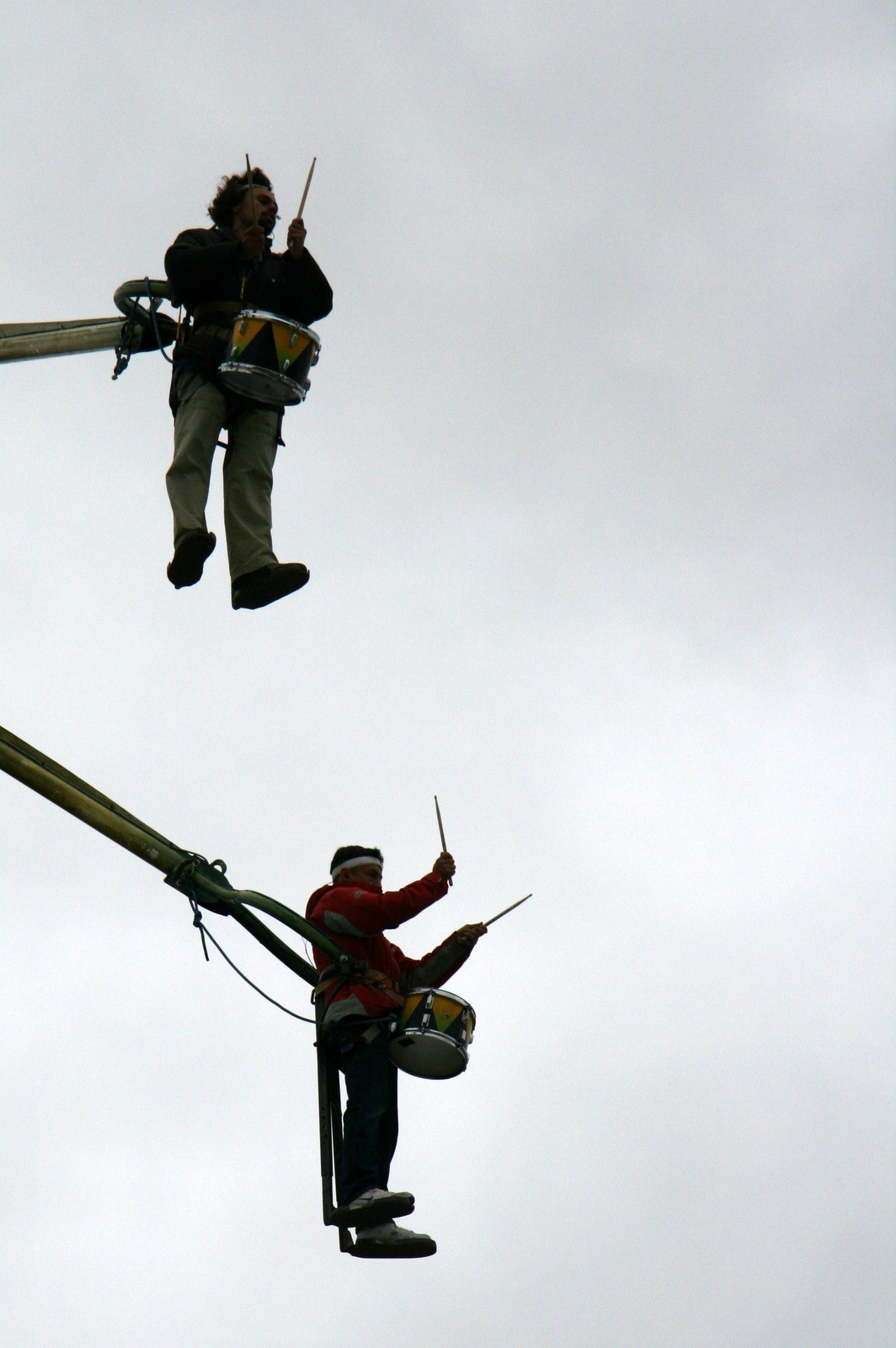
[356,1223,432,1243]
[348,1187,415,1211]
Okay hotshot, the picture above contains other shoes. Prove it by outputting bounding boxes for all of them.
[168,529,212,588]
[230,563,309,609]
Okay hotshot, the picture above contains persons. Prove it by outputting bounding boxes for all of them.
[304,845,488,1260]
[164,168,333,610]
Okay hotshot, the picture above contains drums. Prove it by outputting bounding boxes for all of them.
[394,986,477,1081]
[217,307,322,409]
